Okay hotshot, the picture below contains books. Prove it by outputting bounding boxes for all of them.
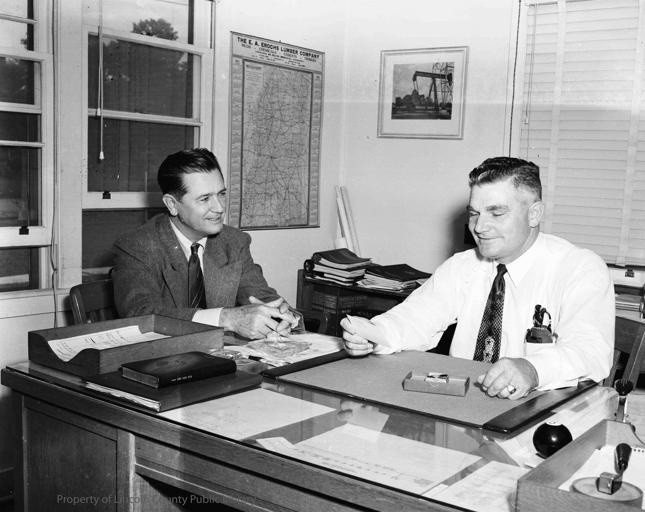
[612,292,645,313]
[85,350,263,414]
[303,246,434,295]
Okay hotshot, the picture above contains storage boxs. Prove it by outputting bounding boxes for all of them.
[25,313,226,378]
[511,421,642,512]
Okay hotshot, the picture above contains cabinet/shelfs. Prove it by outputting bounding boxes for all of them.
[297,266,408,338]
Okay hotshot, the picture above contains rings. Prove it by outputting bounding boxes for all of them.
[506,384,517,396]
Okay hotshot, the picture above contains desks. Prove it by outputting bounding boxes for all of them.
[0,332,643,512]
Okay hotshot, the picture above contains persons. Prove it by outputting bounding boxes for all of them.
[340,156,618,402]
[110,147,306,342]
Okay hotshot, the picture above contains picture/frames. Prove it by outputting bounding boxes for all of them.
[375,45,469,140]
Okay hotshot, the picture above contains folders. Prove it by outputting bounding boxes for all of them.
[304,247,372,286]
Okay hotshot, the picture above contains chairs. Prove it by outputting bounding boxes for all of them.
[605,316,645,390]
[67,277,116,323]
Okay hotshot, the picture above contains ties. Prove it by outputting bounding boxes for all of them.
[188,243,207,309]
[473,263,508,364]
